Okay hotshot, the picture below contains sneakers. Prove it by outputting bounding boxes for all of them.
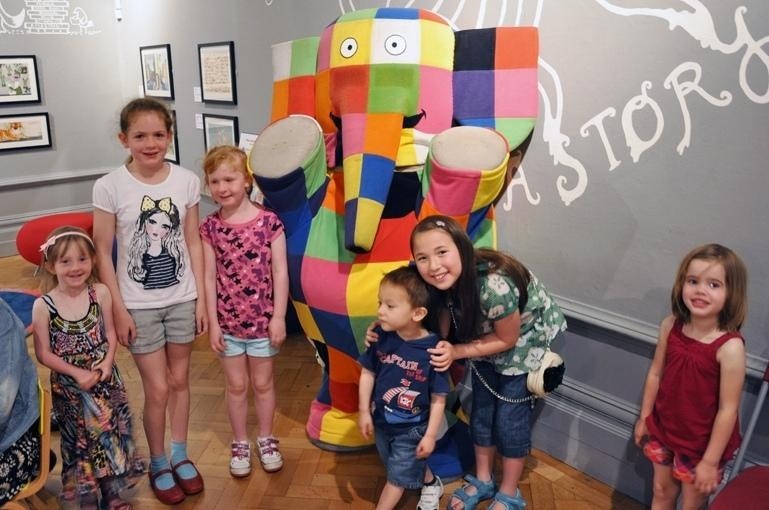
[255,434,284,473]
[229,439,252,477]
[101,495,132,510]
[416,474,444,510]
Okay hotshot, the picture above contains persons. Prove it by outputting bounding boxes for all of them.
[0,297,56,510]
[127,194,186,290]
[634,244,747,510]
[364,215,567,510]
[198,145,289,477]
[93,98,209,504]
[357,267,445,510]
[32,225,147,510]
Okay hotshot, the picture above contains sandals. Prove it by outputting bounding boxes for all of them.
[446,472,498,510]
[488,487,527,510]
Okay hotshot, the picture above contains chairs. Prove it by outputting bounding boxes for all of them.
[1,362,60,510]
[708,359,769,510]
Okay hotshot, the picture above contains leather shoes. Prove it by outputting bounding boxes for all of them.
[170,454,204,495]
[147,460,186,505]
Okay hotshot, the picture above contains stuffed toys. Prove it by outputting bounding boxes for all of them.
[247,8,536,453]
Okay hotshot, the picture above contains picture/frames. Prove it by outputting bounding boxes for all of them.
[0,54,42,106]
[163,111,180,165]
[197,41,237,106]
[202,113,239,154]
[0,112,51,152]
[139,44,175,101]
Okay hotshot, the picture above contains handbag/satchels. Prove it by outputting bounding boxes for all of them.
[525,346,565,397]
